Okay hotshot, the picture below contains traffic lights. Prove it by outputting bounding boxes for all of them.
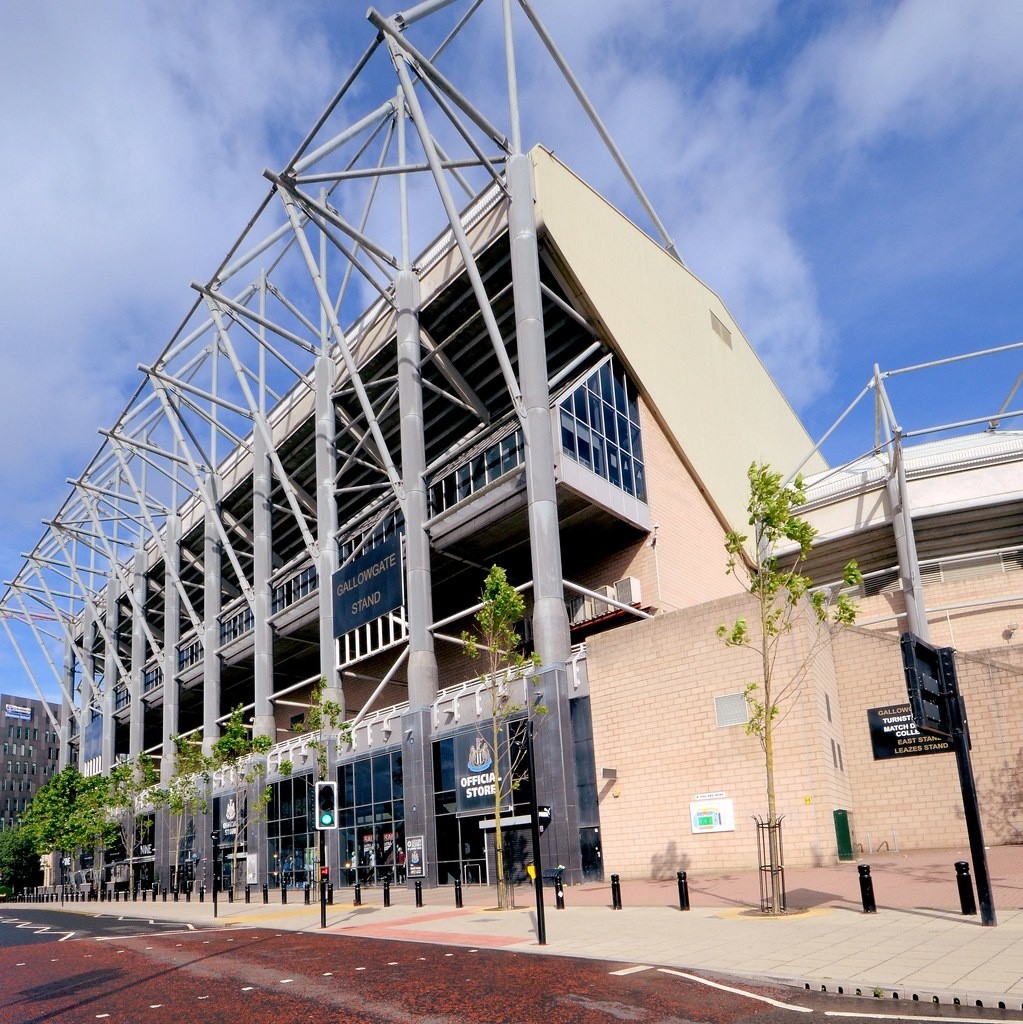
[315,780,337,830]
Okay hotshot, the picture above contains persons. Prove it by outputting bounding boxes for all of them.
[289,856,293,886]
[398,847,406,884]
[282,858,290,878]
[369,851,375,885]
[351,852,359,885]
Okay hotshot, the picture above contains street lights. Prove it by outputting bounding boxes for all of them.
[211,831,220,918]
